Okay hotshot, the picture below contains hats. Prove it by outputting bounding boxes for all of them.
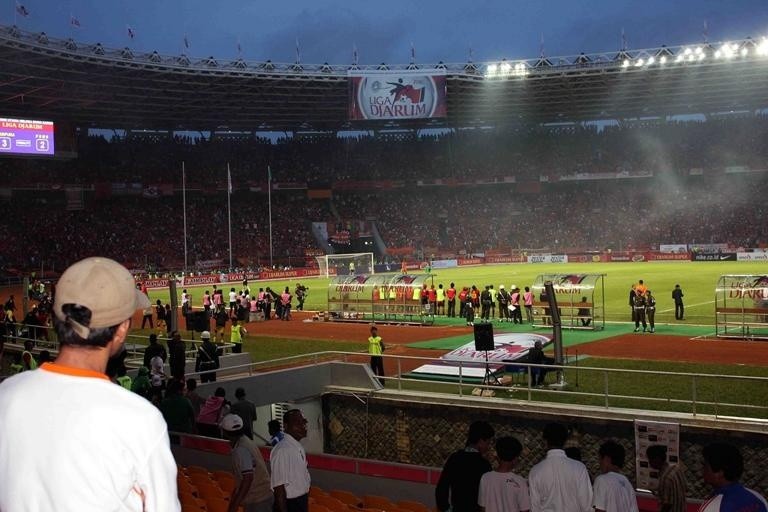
[53,256,153,341]
[218,414,244,432]
[284,408,308,423]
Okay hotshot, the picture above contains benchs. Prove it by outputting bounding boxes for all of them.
[715,306,767,340]
[327,299,429,324]
[532,299,602,329]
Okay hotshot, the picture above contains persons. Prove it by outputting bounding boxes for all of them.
[642,444,688,512]
[268,407,313,511]
[476,433,533,512]
[382,76,408,108]
[0,113,768,449]
[434,419,495,512]
[594,438,640,511]
[0,253,186,512]
[527,421,594,511]
[695,440,768,511]
[214,413,274,511]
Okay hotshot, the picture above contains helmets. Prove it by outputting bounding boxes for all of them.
[201,330,211,339]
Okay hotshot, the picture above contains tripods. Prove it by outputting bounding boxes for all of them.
[480,351,502,396]
[187,331,199,358]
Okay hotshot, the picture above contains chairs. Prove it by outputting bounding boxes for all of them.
[178,463,434,512]
[501,357,525,388]
[528,357,548,388]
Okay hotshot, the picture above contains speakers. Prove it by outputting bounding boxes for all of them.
[194,311,211,331]
[474,323,494,350]
[186,311,194,331]
